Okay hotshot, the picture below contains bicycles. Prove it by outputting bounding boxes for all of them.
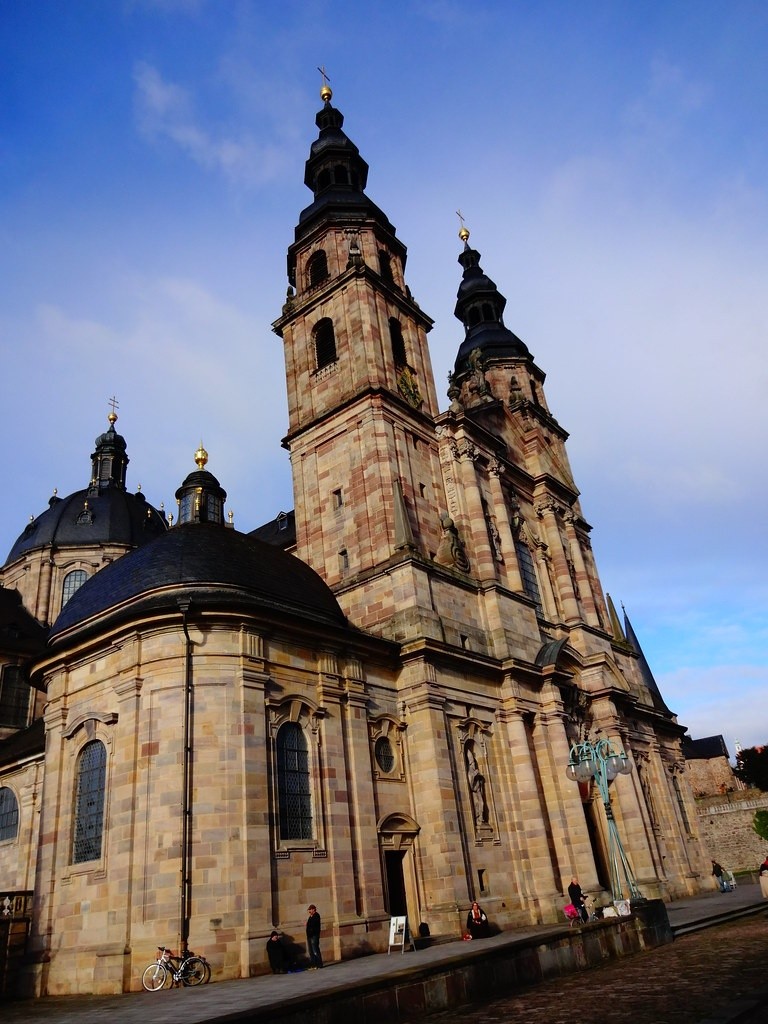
[141,946,207,993]
[569,897,601,929]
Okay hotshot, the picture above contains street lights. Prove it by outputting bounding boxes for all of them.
[565,737,643,902]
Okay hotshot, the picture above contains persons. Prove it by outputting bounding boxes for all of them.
[760,864,768,900]
[764,856,768,865]
[721,867,732,892]
[467,902,487,939]
[306,905,323,969]
[711,860,725,893]
[568,876,588,924]
[719,784,726,793]
[266,931,286,974]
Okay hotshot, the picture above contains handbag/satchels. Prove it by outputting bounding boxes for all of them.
[722,871,731,882]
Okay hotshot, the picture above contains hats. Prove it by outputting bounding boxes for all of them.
[472,902,478,906]
[307,904,316,911]
[271,931,278,939]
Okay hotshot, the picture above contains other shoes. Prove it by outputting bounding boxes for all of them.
[720,889,726,893]
[308,967,320,971]
[294,969,301,972]
[287,969,291,973]
